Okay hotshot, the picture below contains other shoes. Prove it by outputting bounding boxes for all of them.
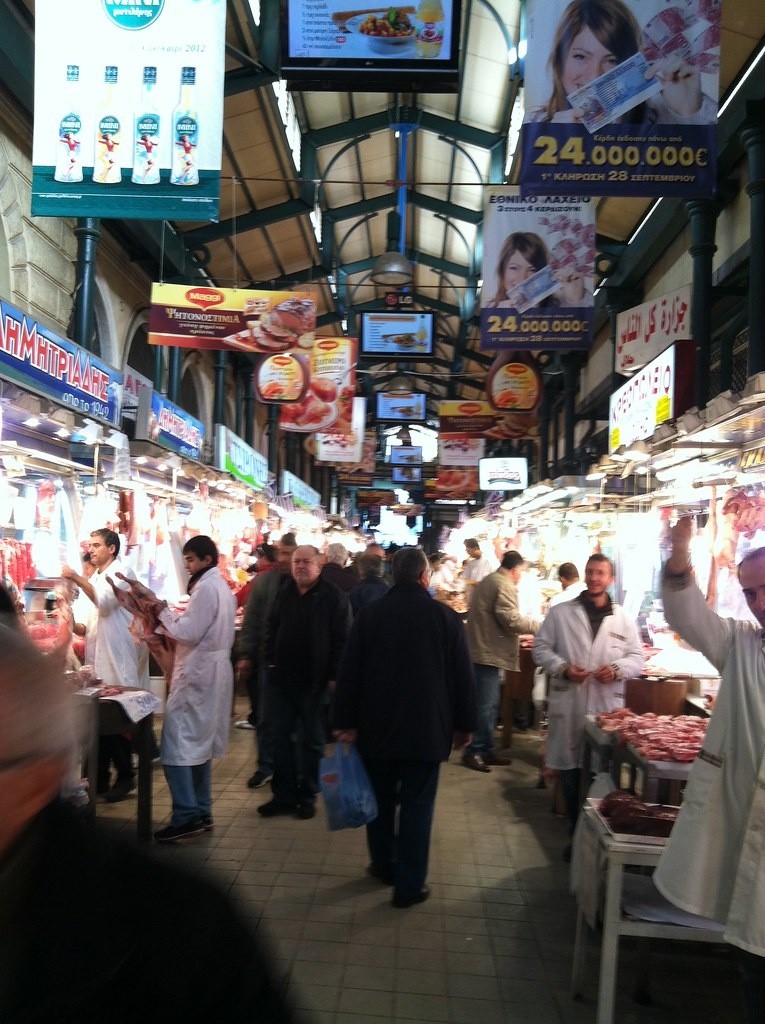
[496,724,528,734]
[257,799,292,815]
[151,746,161,763]
[561,842,573,861]
[370,863,395,885]
[394,885,429,907]
[102,776,136,800]
[296,800,315,818]
[234,720,258,730]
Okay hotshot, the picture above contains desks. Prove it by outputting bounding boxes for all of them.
[68,686,158,842]
[567,715,737,1024]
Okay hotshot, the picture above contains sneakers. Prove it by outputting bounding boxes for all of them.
[247,771,273,788]
[204,812,214,830]
[154,816,204,840]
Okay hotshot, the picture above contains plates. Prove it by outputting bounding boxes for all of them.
[278,393,341,434]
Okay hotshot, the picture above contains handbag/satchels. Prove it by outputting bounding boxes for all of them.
[317,742,379,831]
[532,667,547,711]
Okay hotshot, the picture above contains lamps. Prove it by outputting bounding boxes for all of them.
[369,93,413,283]
[78,423,105,444]
[138,371,414,500]
[626,440,655,459]
[586,462,619,482]
[7,394,43,419]
[106,433,130,449]
[598,454,627,469]
[46,409,76,433]
[612,443,630,462]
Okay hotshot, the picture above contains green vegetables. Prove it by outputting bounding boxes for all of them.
[388,7,408,30]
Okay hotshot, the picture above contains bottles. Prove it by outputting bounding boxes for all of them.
[416,315,428,351]
[53,65,200,185]
[417,0,445,59]
[415,395,422,419]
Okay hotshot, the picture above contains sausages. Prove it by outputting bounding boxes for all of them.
[0,538,37,593]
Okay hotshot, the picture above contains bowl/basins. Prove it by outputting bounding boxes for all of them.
[348,12,419,44]
[387,334,417,351]
[394,407,416,417]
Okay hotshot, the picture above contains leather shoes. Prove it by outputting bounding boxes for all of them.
[483,753,512,764]
[462,753,492,772]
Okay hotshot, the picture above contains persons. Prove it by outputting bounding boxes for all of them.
[550,563,588,608]
[653,516,765,957]
[62,528,160,802]
[462,551,542,772]
[234,532,298,788]
[153,535,236,842]
[482,231,593,308]
[523,0,718,124]
[462,538,501,580]
[329,547,476,906]
[0,620,299,1024]
[533,553,644,862]
[324,542,391,614]
[426,552,467,598]
[256,545,353,818]
